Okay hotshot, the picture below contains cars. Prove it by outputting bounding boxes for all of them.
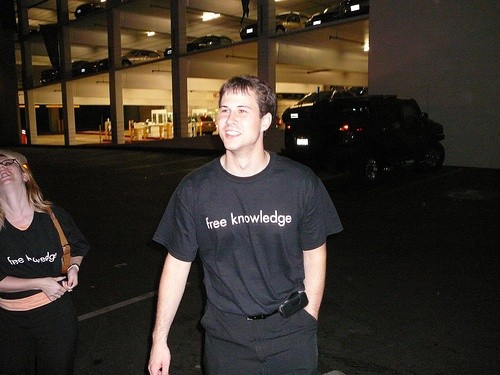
[304,0,370,26]
[93,50,162,70]
[164,35,234,58]
[42,59,90,84]
[81,57,110,74]
[239,11,310,40]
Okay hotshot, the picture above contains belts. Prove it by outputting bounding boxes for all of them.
[244,291,299,320]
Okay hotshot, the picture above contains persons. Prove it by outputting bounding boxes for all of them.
[0,148,91,375]
[145,72,344,375]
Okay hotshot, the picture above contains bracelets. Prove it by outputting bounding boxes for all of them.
[67,263,80,272]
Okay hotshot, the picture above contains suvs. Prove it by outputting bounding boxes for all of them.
[188,116,216,135]
[75,3,105,19]
[285,94,444,179]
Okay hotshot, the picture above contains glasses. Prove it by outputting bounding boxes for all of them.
[0,158,23,171]
